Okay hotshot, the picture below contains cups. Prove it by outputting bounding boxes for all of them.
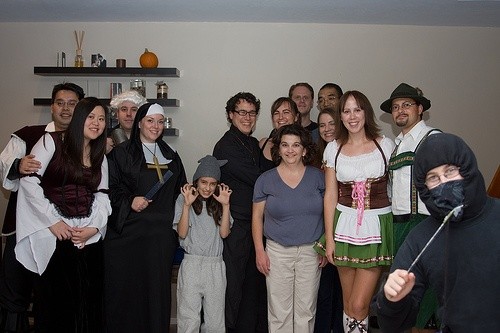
[116,59,126,68]
[163,117,172,129]
[109,83,122,99]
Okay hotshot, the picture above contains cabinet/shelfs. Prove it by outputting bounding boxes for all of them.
[31,66,180,136]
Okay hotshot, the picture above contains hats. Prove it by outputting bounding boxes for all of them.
[193,154,228,182]
[380,83,431,115]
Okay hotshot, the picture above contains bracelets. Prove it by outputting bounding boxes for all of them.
[96,228,99,232]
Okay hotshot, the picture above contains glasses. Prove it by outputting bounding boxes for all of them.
[424,166,461,188]
[54,100,76,108]
[390,102,416,112]
[234,110,258,117]
[317,96,336,106]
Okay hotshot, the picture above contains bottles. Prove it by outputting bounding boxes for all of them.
[157,83,168,100]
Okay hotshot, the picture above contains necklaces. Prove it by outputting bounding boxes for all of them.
[142,142,168,182]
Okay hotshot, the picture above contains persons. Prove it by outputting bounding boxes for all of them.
[172,155,234,333]
[251,123,329,333]
[0,82,188,333]
[313,91,393,333]
[380,84,443,333]
[270,82,347,333]
[375,132,500,333]
[212,92,274,333]
